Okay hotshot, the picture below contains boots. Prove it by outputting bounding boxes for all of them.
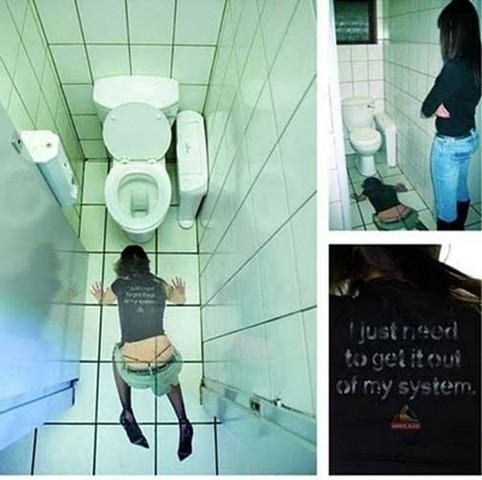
[436,198,470,230]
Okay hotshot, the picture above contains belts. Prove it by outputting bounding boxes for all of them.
[129,358,175,376]
[437,127,475,141]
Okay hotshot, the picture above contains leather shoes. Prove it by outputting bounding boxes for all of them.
[178,419,193,461]
[119,409,149,448]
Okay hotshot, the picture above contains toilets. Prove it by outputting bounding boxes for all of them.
[342,95,383,175]
[91,75,179,243]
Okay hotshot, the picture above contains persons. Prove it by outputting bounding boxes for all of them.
[89,245,195,464]
[416,2,481,230]
[348,173,428,234]
[397,263,481,408]
[4,242,82,397]
